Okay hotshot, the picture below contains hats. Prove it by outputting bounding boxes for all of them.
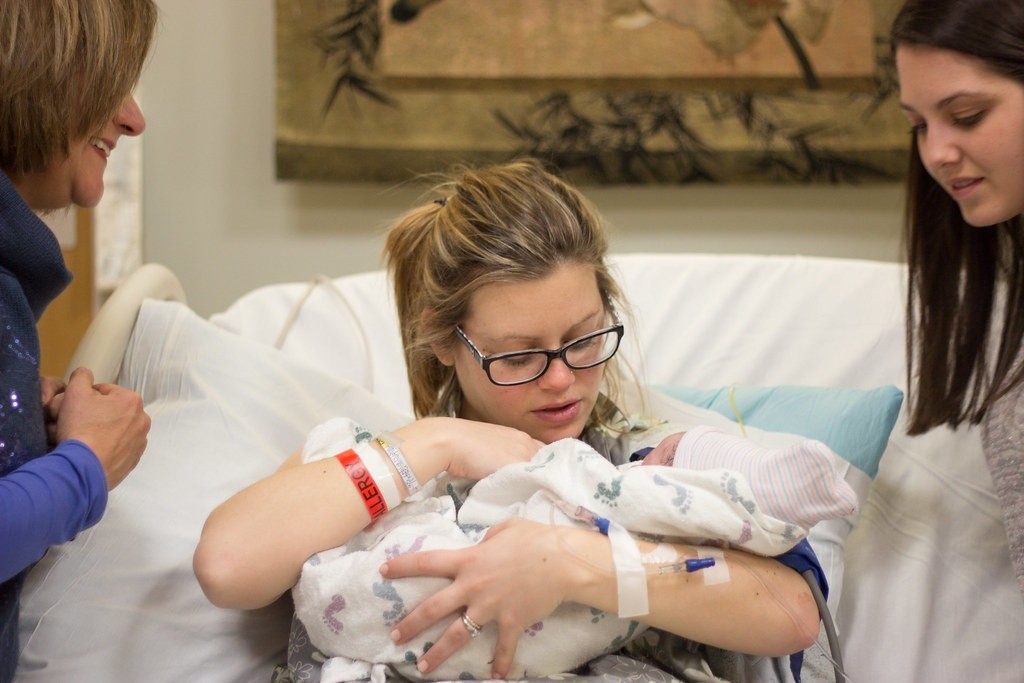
[672,421,859,532]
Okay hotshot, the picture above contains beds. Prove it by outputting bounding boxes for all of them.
[10,253,908,681]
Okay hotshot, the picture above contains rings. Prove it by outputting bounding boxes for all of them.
[462,612,481,638]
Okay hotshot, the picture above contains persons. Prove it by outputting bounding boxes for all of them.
[0,0,161,683]
[285,416,859,682]
[192,155,830,683]
[888,0,1024,583]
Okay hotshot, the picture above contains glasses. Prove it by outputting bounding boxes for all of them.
[452,296,624,386]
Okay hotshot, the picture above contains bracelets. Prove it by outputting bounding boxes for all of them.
[335,441,401,520]
[377,430,423,495]
